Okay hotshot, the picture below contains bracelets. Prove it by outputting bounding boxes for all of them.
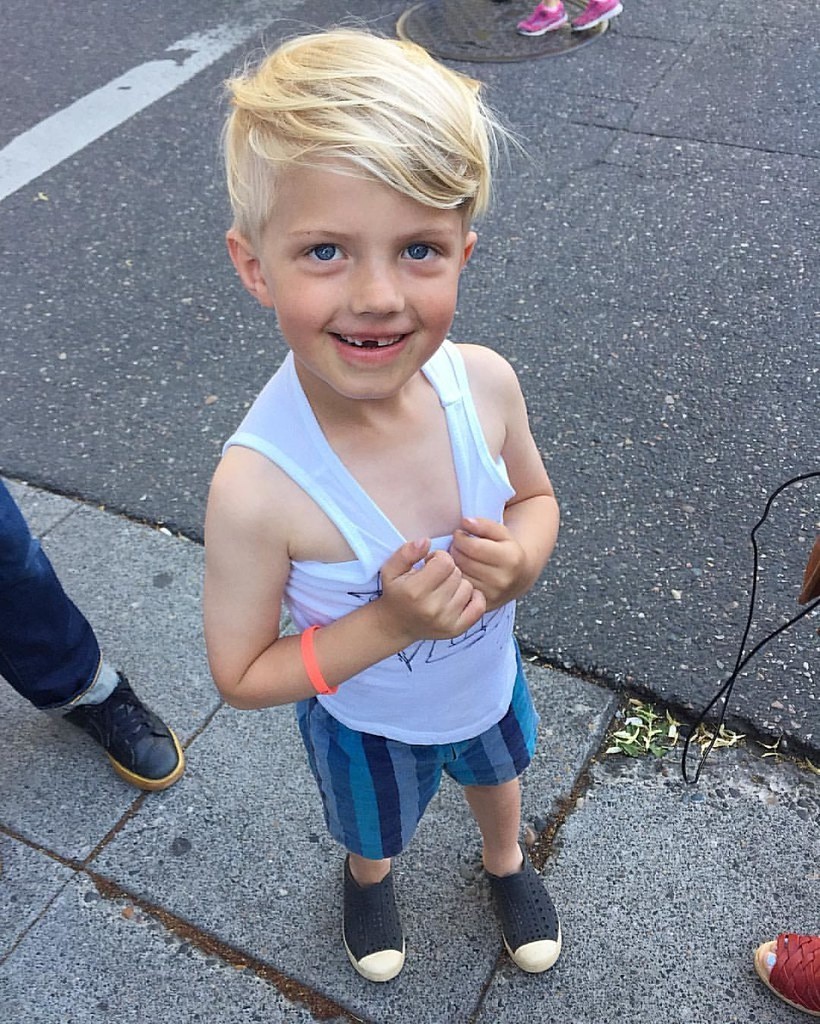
[301,625,339,695]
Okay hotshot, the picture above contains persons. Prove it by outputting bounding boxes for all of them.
[0,477,184,793]
[203,28,561,982]
[517,1,624,36]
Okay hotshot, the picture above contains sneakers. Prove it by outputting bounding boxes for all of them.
[571,0,623,32]
[59,670,185,790]
[516,3,567,37]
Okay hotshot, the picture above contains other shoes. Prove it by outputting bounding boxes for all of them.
[755,931,820,1018]
[484,843,561,974]
[341,854,405,982]
